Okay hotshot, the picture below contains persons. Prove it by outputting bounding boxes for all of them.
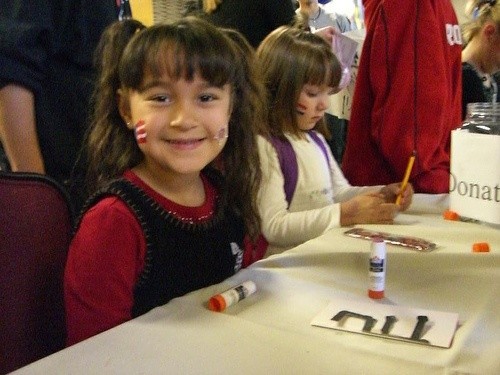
[248,25,413,262]
[1,0,132,179]
[452,1,500,123]
[64,19,266,345]
[341,2,462,194]
[294,0,353,162]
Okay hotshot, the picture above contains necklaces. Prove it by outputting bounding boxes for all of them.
[309,7,321,21]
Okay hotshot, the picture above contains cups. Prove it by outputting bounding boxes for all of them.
[449,102,500,228]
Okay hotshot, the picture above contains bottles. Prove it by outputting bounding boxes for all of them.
[117,0,133,20]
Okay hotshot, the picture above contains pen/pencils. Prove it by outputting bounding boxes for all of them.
[395,150,417,205]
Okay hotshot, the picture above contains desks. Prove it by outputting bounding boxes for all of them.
[9,213,500,375]
